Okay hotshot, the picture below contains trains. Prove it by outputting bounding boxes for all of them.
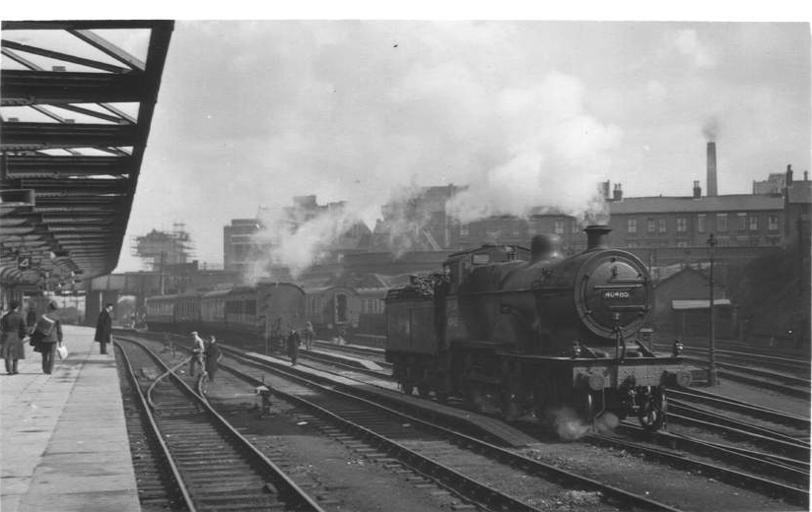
[383,221,706,438]
[141,281,310,352]
[302,284,364,341]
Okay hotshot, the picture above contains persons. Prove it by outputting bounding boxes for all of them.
[94,302,116,355]
[187,330,205,377]
[25,306,38,336]
[304,320,314,351]
[285,328,303,368]
[202,335,220,383]
[0,301,25,375]
[31,301,63,374]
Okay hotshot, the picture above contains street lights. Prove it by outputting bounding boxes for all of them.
[794,210,806,350]
[705,230,719,388]
